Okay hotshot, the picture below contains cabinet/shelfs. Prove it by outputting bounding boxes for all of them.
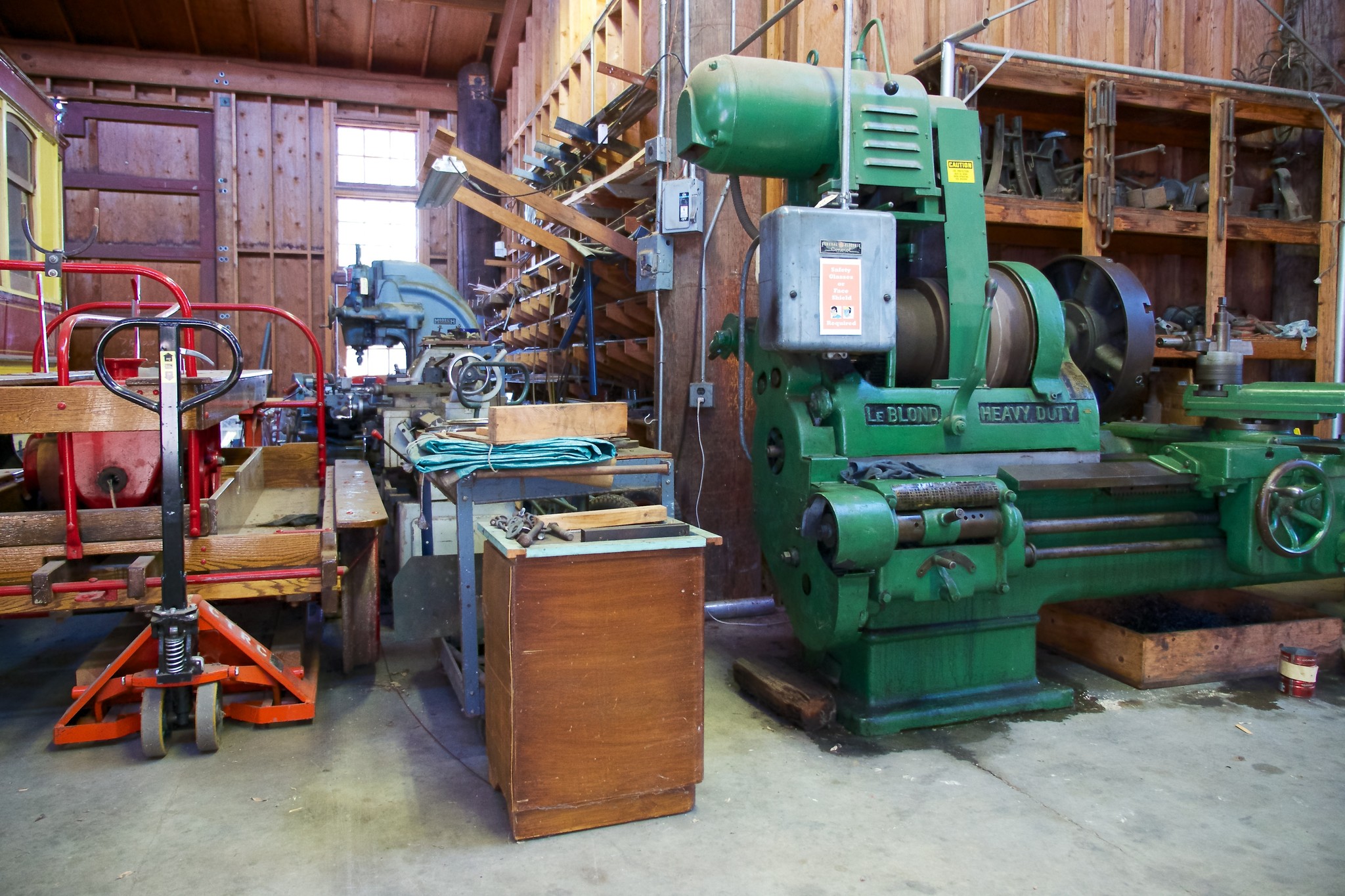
[908,53,1345,440]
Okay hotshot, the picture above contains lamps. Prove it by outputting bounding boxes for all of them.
[415,156,467,210]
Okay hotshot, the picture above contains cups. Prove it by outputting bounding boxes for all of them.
[1278,646,1318,697]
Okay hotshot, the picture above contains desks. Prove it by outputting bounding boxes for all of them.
[416,428,676,719]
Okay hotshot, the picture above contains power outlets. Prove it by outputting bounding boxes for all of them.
[689,382,716,408]
